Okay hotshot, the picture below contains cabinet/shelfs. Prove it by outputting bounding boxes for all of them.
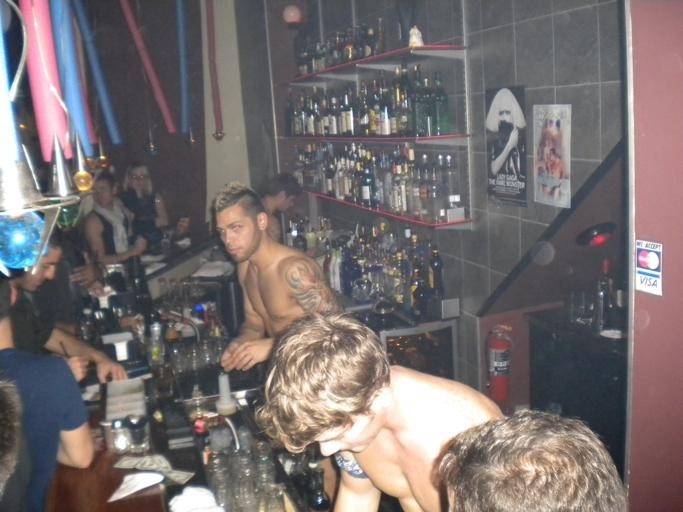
[289,43,476,233]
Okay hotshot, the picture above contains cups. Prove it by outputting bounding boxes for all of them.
[567,292,594,326]
[207,439,329,512]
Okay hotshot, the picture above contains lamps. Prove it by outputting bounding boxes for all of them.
[283,4,318,36]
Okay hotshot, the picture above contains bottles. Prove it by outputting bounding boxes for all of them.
[595,257,628,330]
[100,410,167,453]
[74,280,226,398]
[192,418,210,465]
[279,18,458,319]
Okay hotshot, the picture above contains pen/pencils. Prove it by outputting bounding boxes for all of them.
[59,341,70,360]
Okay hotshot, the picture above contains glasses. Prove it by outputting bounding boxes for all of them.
[129,175,145,180]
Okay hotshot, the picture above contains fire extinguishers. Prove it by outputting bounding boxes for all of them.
[486,323,513,402]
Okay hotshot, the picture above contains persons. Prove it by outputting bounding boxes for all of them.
[210,178,346,372]
[538,119,561,199]
[251,312,504,511]
[486,88,525,183]
[429,407,629,512]
[259,171,302,241]
